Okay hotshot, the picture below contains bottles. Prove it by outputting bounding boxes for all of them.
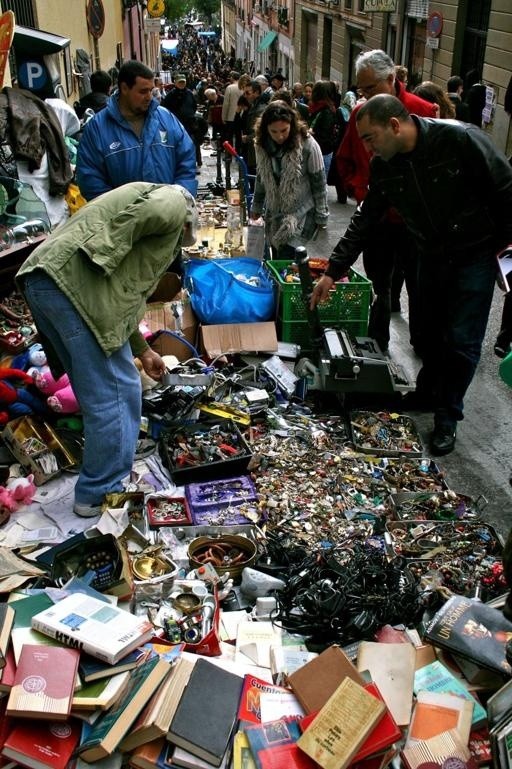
[2,217,49,249]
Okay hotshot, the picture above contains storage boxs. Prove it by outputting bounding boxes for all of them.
[138,287,196,365]
[2,414,75,487]
[196,322,278,364]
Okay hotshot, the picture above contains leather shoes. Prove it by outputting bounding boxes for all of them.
[430,428,454,456]
[404,390,423,411]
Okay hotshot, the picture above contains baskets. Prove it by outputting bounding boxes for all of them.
[264,256,372,351]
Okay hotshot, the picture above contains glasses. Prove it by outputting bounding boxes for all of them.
[357,83,377,95]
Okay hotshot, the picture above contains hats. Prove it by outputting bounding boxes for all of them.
[175,74,186,83]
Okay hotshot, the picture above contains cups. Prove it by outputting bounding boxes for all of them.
[207,221,215,243]
[195,222,208,246]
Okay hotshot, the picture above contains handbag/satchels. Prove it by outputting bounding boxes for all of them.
[183,257,274,324]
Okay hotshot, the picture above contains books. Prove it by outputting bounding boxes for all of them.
[229,593,511,768]
[0,530,247,769]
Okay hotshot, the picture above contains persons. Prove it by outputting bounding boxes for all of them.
[494,289,512,358]
[309,93,511,456]
[14,181,197,518]
[75,66,120,121]
[75,60,198,300]
[309,50,486,359]
[151,26,318,194]
[248,96,328,261]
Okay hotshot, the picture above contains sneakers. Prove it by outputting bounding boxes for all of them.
[73,482,156,517]
[134,438,156,458]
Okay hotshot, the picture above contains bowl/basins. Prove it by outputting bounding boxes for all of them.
[189,534,258,580]
[174,593,203,612]
[132,555,167,581]
